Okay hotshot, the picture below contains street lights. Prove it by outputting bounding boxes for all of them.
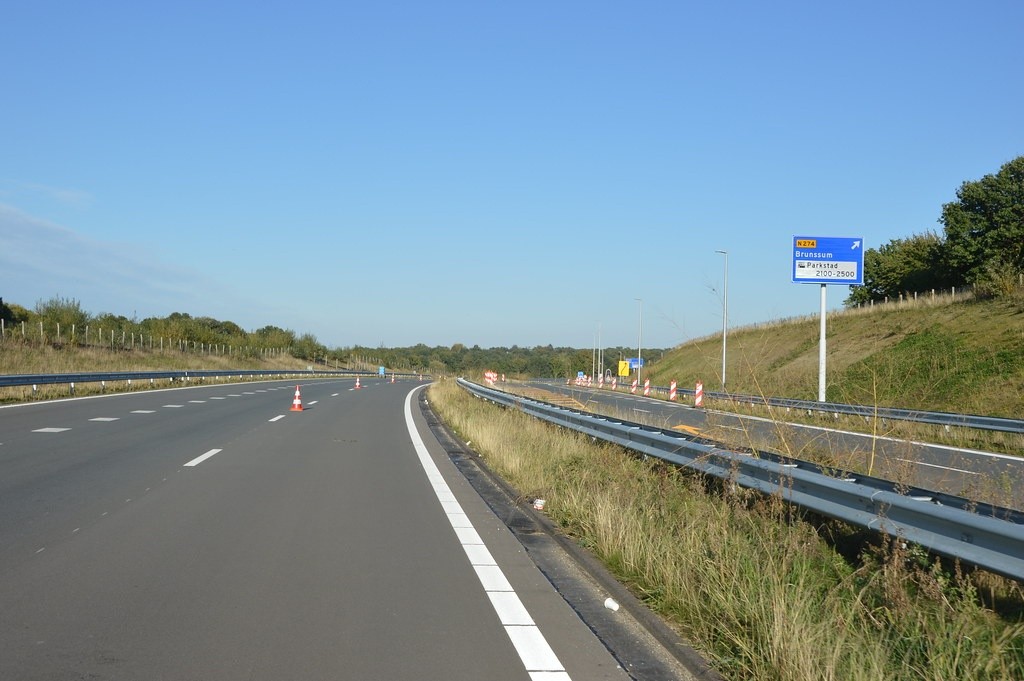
[592,320,605,382]
[714,249,729,388]
[635,298,642,386]
[618,350,622,384]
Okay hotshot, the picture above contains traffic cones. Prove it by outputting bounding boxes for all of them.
[389,372,396,383]
[353,375,362,388]
[289,385,303,411]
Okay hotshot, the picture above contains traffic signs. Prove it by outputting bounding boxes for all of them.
[792,237,866,285]
